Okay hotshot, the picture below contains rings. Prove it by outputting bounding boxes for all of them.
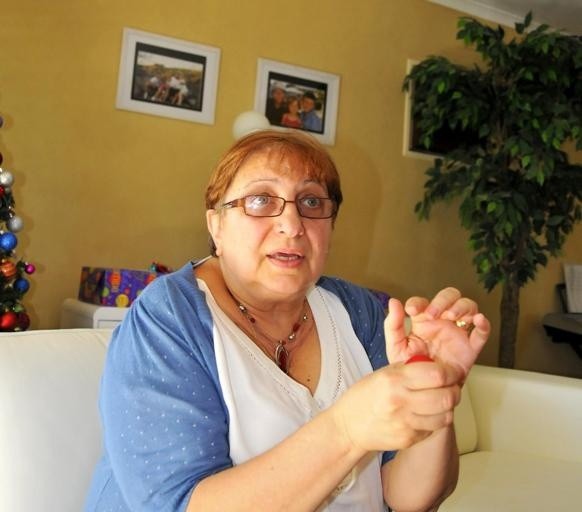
[455,317,472,331]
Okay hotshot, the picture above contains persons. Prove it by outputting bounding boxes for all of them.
[83,132,494,511]
[299,90,321,132]
[280,95,302,129]
[267,80,288,126]
[143,70,201,106]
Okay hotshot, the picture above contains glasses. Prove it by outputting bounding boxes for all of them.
[221,192,339,221]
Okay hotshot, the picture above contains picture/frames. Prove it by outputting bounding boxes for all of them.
[117,29,221,127]
[403,59,476,168]
[253,56,340,146]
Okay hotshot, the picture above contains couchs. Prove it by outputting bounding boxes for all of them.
[0,323,582,512]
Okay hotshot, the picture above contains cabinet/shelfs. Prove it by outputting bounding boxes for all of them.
[61,297,129,329]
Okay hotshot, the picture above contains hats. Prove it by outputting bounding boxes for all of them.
[271,81,289,93]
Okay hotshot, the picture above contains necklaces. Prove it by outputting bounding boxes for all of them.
[235,301,311,375]
